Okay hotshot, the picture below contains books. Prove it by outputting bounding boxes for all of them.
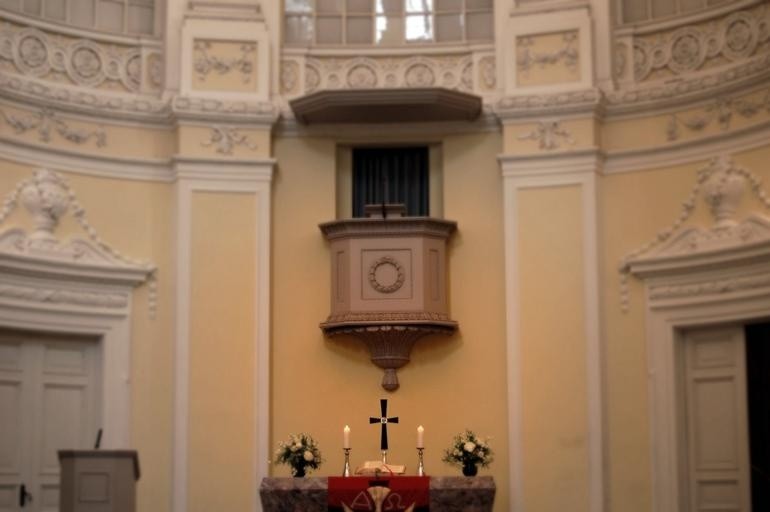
[353,460,408,475]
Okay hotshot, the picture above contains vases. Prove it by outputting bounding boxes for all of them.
[463,461,478,476]
[293,463,306,477]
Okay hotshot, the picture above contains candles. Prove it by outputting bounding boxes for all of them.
[416,424,424,448]
[344,425,351,448]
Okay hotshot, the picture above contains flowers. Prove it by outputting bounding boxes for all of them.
[441,428,496,472]
[265,431,327,477]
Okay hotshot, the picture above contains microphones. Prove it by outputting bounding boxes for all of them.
[94,427,106,448]
